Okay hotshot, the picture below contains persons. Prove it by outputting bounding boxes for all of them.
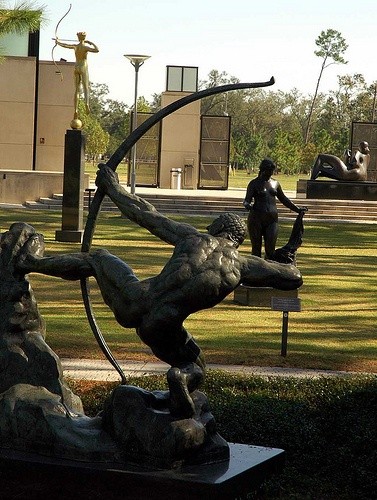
[311,141,371,182]
[55,32,99,121]
[243,159,309,260]
[22,164,302,415]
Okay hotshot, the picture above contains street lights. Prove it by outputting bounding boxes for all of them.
[123,54,151,194]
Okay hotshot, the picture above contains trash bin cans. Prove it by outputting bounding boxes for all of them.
[169,168,182,189]
